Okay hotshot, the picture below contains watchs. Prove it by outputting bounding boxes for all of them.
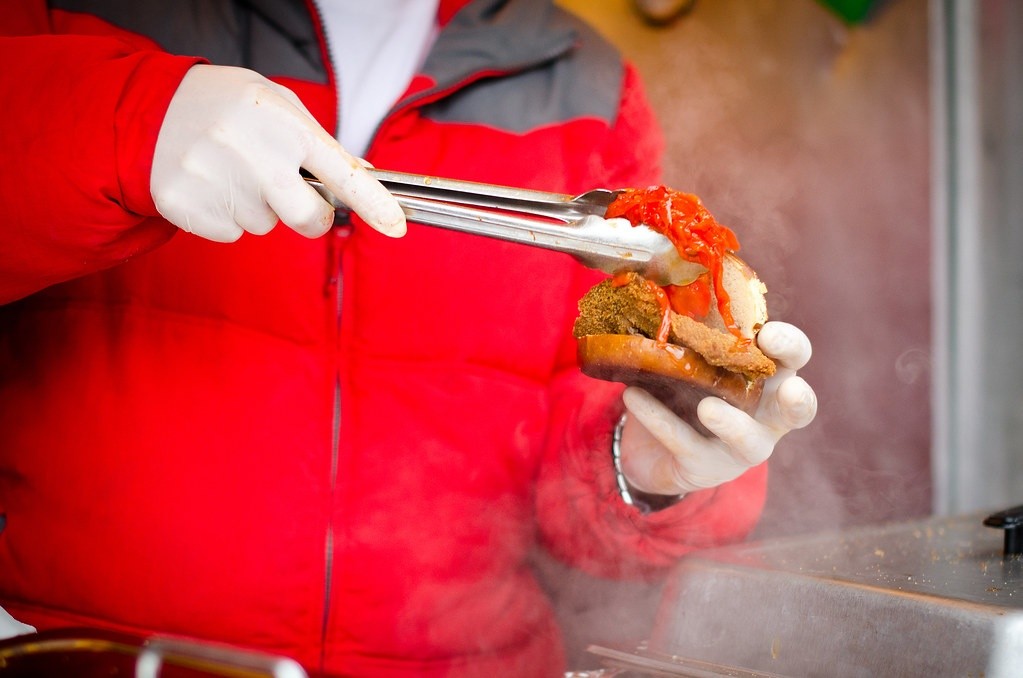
[612,412,686,517]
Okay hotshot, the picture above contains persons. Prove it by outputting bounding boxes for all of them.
[0,0,822,677]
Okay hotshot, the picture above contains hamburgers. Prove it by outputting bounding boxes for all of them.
[570,184,779,442]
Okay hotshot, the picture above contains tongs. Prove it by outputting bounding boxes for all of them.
[299,160,707,288]
